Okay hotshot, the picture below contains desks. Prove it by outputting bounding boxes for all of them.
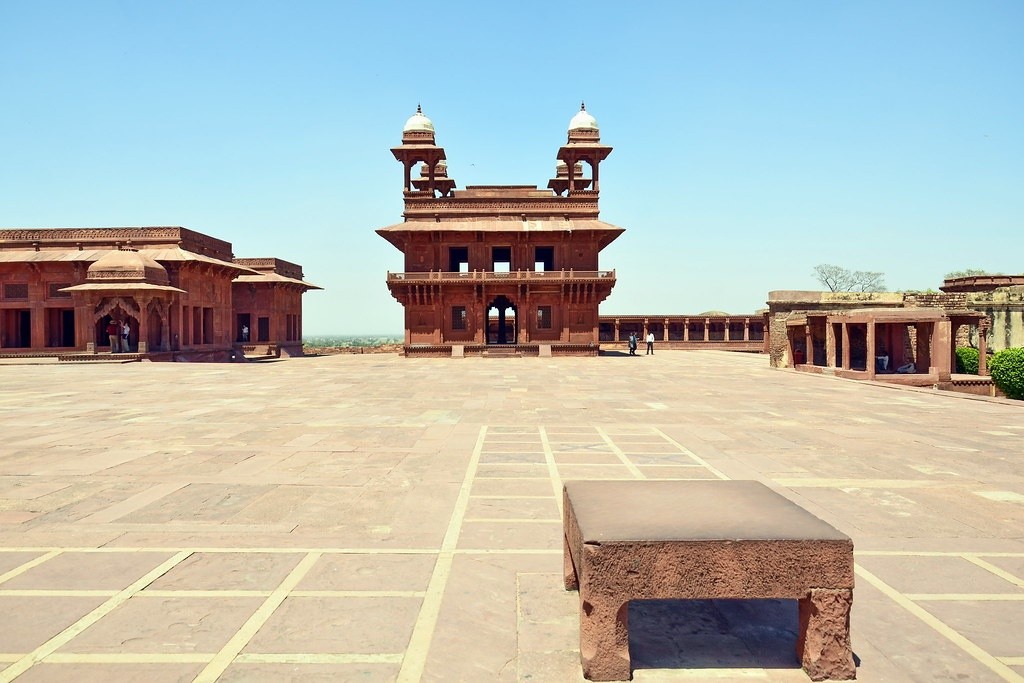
[561,477,859,683]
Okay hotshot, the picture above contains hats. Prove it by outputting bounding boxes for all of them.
[110,320,116,325]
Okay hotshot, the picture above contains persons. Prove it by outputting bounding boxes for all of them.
[851,352,914,373]
[628,331,640,356]
[242,324,248,342]
[106,320,130,353]
[645,330,655,355]
